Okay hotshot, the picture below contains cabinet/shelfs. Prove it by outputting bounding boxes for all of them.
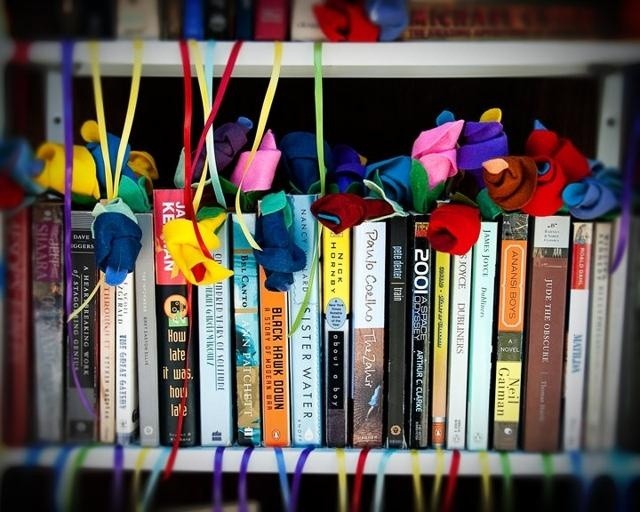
[0,35,640,477]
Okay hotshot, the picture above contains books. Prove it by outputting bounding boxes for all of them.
[2,1,639,40]
[0,192,640,455]
